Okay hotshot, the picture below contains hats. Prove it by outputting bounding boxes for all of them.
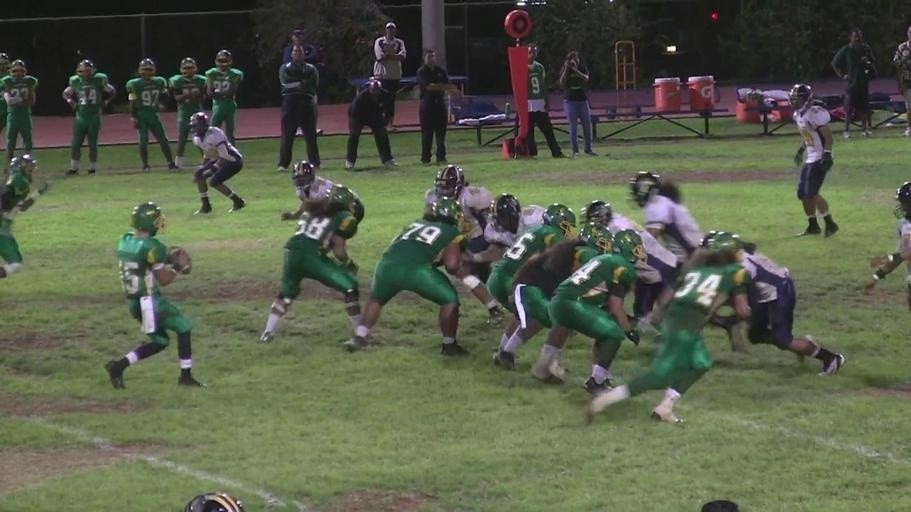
[290,29,304,35]
[386,22,397,29]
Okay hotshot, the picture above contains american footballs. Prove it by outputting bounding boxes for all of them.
[167,246,191,274]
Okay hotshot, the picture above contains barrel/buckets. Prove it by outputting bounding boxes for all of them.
[653,77,683,113]
[686,75,717,110]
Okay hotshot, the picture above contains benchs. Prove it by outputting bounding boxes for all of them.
[466,88,911,150]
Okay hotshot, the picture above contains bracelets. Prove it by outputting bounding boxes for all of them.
[872,268,885,281]
[888,253,901,264]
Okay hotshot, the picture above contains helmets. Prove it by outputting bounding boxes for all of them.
[188,112,210,138]
[327,183,354,211]
[428,194,465,229]
[6,154,38,183]
[787,81,815,115]
[539,167,741,264]
[0,48,235,77]
[433,162,466,202]
[127,200,166,238]
[895,179,910,226]
[491,192,523,235]
[291,160,317,192]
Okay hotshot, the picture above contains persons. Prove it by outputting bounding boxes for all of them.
[788,84,839,237]
[416,49,457,165]
[526,42,565,158]
[893,25,911,136]
[104,202,208,391]
[0,154,53,280]
[866,181,911,314]
[830,27,878,138]
[373,22,407,131]
[702,500,739,512]
[345,77,401,170]
[282,27,326,137]
[558,50,599,159]
[277,43,322,172]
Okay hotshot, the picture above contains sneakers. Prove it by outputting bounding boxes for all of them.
[192,204,212,217]
[227,195,248,213]
[439,340,472,359]
[820,223,840,239]
[794,224,820,240]
[840,126,911,140]
[341,337,363,353]
[479,304,688,432]
[361,333,383,345]
[549,149,598,162]
[276,125,452,172]
[63,162,183,176]
[176,375,209,390]
[101,358,128,393]
[816,355,845,379]
